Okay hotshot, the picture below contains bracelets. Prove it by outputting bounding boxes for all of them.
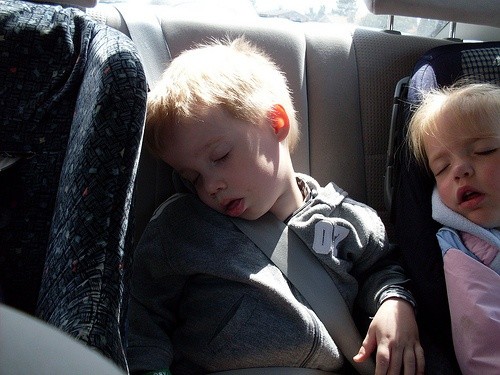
[374,285,415,309]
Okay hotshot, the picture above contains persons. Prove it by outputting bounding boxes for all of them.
[406,82,500,375]
[117,36,426,375]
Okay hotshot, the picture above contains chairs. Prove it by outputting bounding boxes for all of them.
[0,0,146,375]
[306,1,500,375]
[160,12,309,375]
